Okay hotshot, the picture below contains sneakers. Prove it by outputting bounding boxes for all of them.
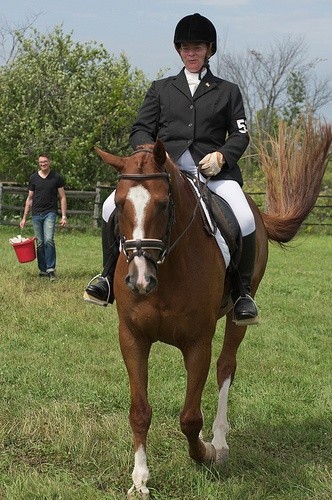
[49,273,56,281]
[39,271,47,276]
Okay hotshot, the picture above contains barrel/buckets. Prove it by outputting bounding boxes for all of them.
[10,227,36,263]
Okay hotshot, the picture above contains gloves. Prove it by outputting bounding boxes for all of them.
[197,151,225,176]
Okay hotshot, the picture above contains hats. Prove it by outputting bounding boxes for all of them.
[174,13,217,53]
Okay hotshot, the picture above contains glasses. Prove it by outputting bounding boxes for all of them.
[179,46,208,52]
[39,160,48,164]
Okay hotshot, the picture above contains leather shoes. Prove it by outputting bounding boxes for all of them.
[86,275,115,304]
[235,298,257,319]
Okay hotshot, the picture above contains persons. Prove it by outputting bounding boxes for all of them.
[86,12,258,320]
[19,153,68,282]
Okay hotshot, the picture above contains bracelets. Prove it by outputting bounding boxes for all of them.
[62,215,68,219]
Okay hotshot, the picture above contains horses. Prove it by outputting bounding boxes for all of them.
[94,103,332,500]
